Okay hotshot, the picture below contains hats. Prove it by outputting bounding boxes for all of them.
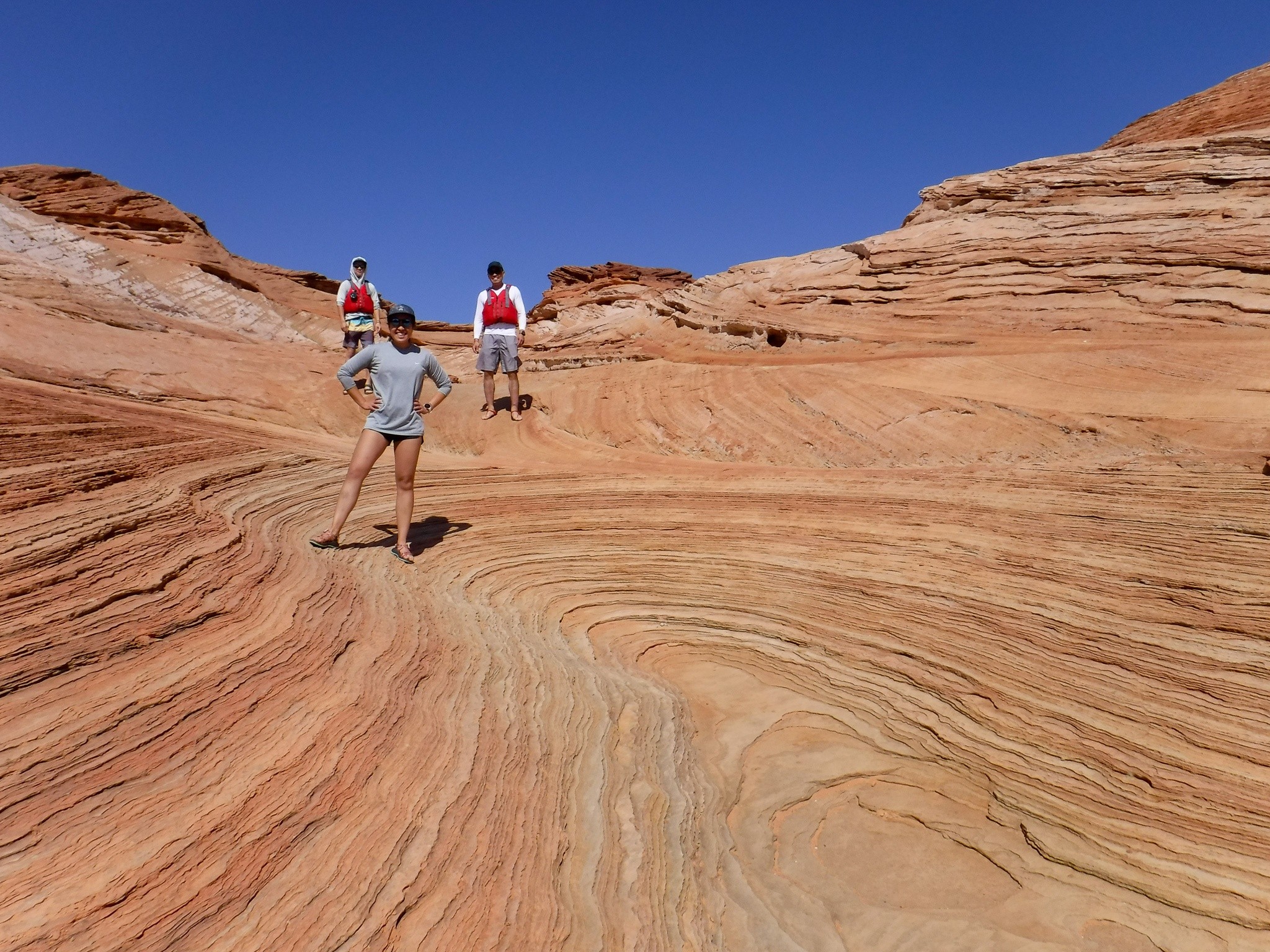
[488,261,503,270]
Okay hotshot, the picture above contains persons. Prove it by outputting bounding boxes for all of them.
[310,304,452,564]
[336,257,381,394]
[471,262,526,420]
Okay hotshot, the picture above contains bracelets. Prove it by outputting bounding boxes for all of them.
[376,319,381,324]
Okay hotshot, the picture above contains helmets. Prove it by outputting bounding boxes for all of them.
[387,305,416,324]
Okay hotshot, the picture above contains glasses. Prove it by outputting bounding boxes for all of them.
[488,269,502,275]
[389,319,412,326]
[354,264,364,268]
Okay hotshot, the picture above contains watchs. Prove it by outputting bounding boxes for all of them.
[519,331,526,336]
[424,403,432,412]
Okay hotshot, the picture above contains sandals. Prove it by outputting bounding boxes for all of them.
[391,544,414,563]
[309,529,339,548]
[364,384,373,394]
[343,388,348,394]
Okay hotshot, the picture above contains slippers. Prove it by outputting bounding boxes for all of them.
[482,410,497,420]
[511,410,522,421]
